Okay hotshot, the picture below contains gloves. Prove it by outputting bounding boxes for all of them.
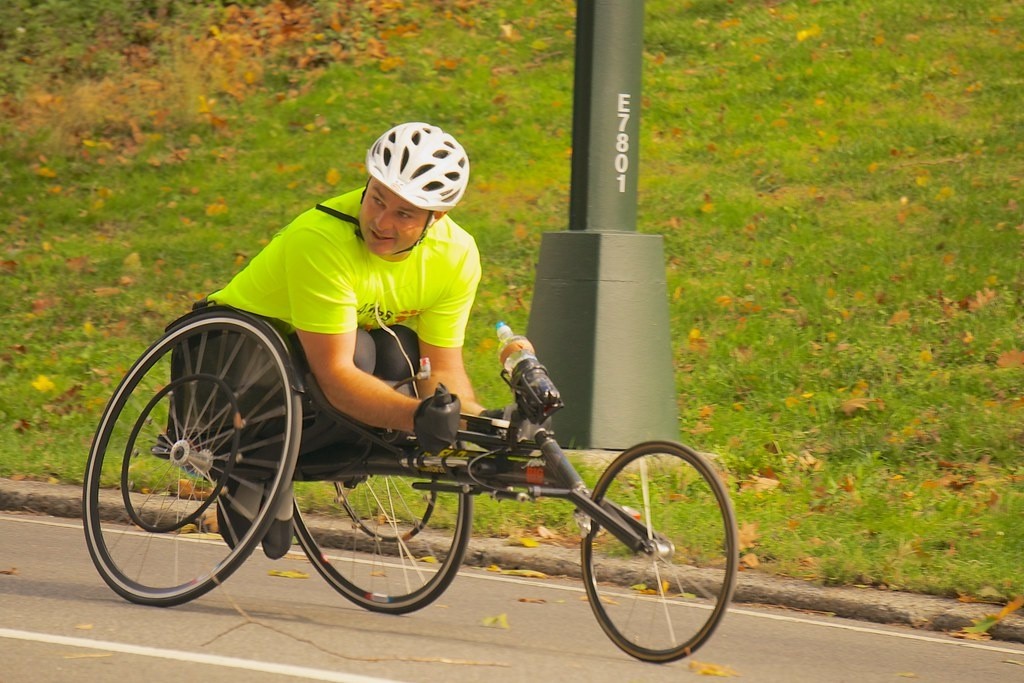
[414,394,461,456]
[468,408,519,450]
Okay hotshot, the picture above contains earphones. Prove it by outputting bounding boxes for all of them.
[428,214,435,227]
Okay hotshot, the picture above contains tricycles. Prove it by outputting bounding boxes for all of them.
[81,302,741,665]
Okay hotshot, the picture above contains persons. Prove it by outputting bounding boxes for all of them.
[190,121,516,560]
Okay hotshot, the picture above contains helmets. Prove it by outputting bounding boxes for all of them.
[365,120,470,210]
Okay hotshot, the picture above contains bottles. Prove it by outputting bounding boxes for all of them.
[496,323,561,412]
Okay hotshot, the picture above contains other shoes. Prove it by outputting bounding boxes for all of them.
[216,478,294,559]
